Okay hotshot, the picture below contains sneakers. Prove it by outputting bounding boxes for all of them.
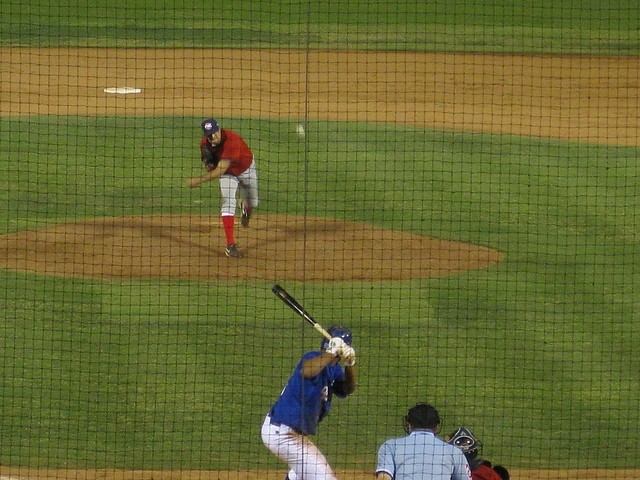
[225,244,241,258]
[239,203,253,227]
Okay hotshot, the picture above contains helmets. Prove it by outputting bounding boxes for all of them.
[402,401,444,435]
[444,426,484,469]
[322,326,352,361]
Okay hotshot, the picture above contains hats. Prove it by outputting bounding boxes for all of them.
[201,118,219,136]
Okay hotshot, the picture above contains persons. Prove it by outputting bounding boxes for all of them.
[186,118,259,259]
[260,324,356,480]
[375,404,473,480]
[447,426,509,480]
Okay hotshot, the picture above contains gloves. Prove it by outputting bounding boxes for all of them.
[340,342,356,367]
[325,337,344,357]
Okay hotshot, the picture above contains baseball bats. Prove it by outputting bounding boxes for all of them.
[271,283,356,364]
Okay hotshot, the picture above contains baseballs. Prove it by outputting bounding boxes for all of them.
[296,123,306,138]
